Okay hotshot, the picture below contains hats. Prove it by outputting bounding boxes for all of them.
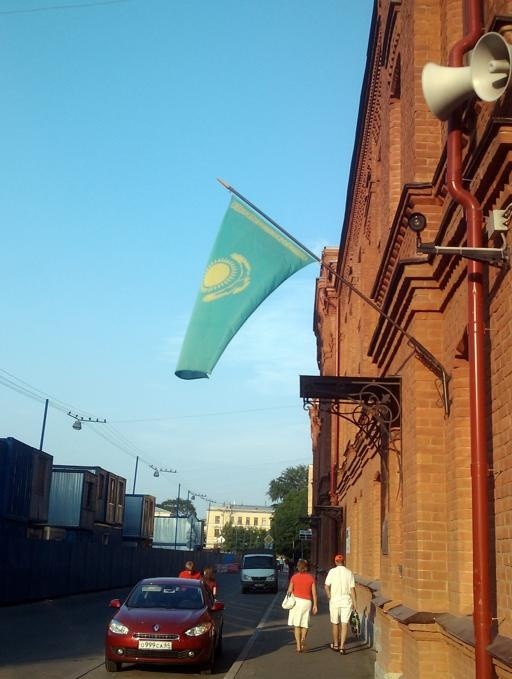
[335,555,345,561]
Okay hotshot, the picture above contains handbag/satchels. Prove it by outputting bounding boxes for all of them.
[282,591,297,610]
[349,610,361,642]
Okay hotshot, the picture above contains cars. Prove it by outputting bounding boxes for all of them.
[104,577,224,675]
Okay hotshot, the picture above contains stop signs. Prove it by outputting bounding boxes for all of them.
[285,559,289,564]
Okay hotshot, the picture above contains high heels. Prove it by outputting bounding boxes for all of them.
[295,642,306,656]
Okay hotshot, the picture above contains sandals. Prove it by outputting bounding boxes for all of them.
[339,648,345,655]
[330,642,339,652]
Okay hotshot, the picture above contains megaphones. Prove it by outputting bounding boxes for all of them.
[421,61,476,122]
[470,31,512,103]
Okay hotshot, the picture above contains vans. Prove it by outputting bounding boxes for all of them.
[241,554,278,594]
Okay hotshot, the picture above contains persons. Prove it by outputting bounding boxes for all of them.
[202,566,217,598]
[281,558,318,652]
[278,556,284,573]
[323,554,361,655]
[177,560,202,592]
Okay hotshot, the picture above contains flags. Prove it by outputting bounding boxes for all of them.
[174,194,318,382]
